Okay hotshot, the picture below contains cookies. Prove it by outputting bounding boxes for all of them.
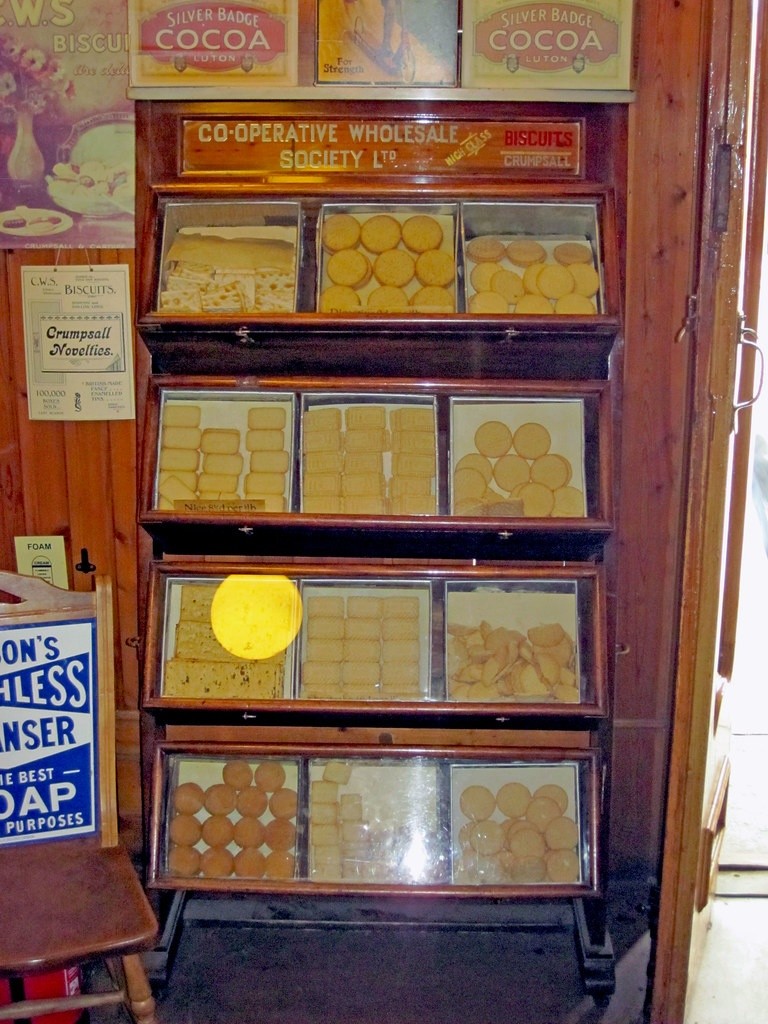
[152,214,602,519]
[162,584,582,885]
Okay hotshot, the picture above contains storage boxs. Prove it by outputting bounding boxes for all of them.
[147,197,614,893]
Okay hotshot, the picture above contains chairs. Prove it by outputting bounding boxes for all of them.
[0,563,161,1024]
